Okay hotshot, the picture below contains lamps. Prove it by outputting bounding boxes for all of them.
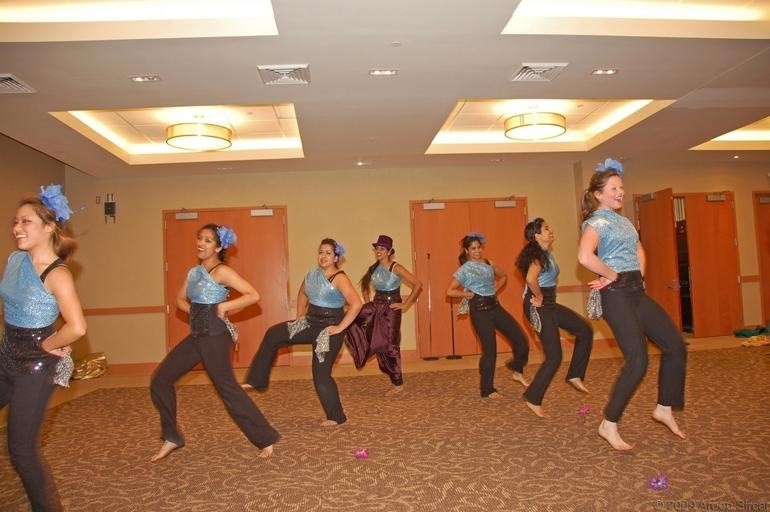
[165,122,232,152]
[505,110,565,140]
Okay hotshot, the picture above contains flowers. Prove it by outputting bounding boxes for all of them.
[651,474,669,493]
[39,182,85,223]
[352,448,370,459]
[468,230,486,244]
[217,224,239,249]
[578,404,592,415]
[335,242,345,257]
[594,157,625,175]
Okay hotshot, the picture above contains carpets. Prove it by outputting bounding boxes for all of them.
[0,343,770,511]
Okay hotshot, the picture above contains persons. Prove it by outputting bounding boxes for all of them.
[575,158,688,459]
[241,238,364,434]
[148,222,281,465]
[516,217,594,421]
[0,182,88,511]
[345,235,423,397]
[445,228,532,399]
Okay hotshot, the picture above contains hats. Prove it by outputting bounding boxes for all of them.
[372,235,395,256]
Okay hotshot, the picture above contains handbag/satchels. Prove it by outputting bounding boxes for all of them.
[72,352,109,380]
